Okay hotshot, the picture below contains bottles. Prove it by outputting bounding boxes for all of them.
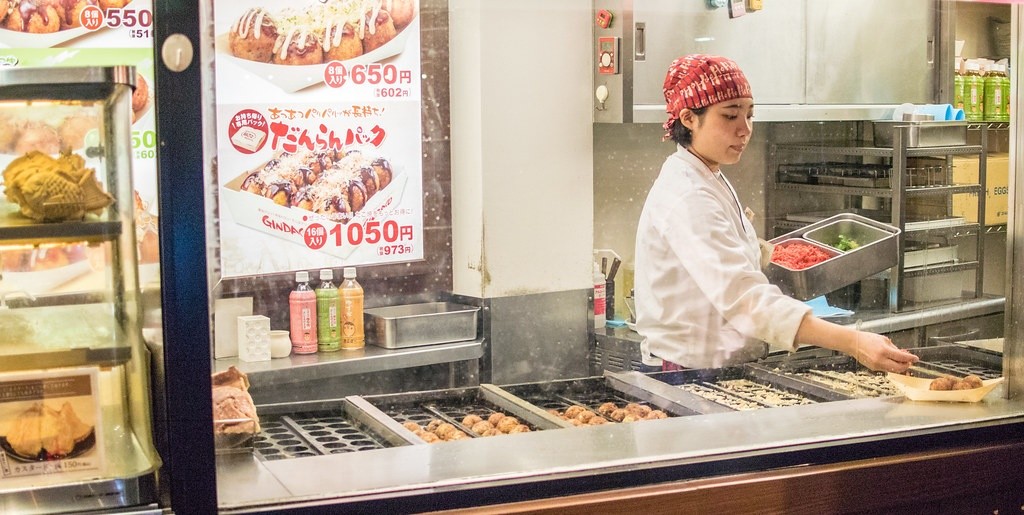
[953,62,1010,121]
[290,267,365,355]
[592,264,607,329]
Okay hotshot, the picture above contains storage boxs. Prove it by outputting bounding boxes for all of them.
[885,151,1009,225]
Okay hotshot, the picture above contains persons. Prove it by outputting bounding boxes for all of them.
[633,56,919,374]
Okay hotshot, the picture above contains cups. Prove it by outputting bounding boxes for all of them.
[270,330,292,358]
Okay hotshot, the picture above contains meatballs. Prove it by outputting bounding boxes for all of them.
[229,0,414,65]
[402,401,667,444]
[240,151,392,223]
[0,0,132,34]
[928,374,984,391]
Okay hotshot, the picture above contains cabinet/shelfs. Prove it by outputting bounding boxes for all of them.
[0,65,164,515]
[584,293,1006,374]
[764,120,988,314]
[592,0,955,123]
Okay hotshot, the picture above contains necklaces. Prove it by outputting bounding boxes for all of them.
[682,145,748,236]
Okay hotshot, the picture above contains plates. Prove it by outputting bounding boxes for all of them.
[0,430,95,462]
